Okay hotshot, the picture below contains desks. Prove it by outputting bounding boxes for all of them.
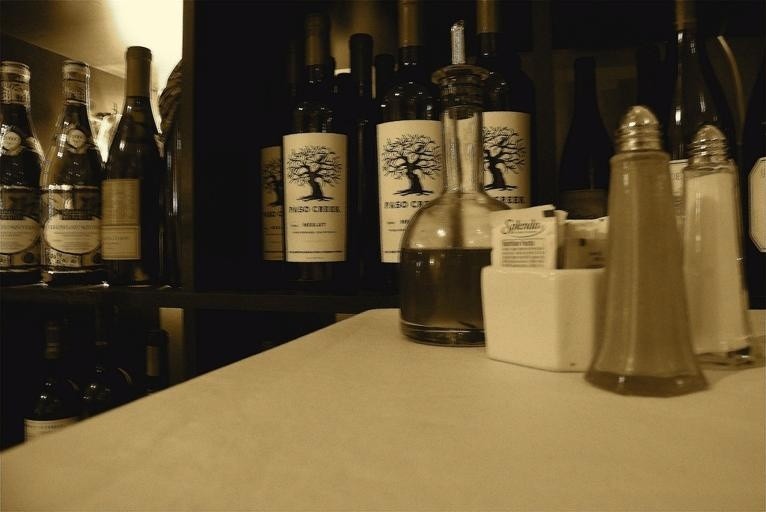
[0,304,764,512]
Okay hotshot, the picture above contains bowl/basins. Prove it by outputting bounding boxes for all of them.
[476,264,609,377]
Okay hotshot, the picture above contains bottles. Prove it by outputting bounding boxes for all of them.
[22,324,169,443]
[1,59,43,287]
[584,106,713,399]
[675,121,765,375]
[245,0,739,297]
[99,44,170,288]
[396,65,516,350]
[39,58,106,287]
